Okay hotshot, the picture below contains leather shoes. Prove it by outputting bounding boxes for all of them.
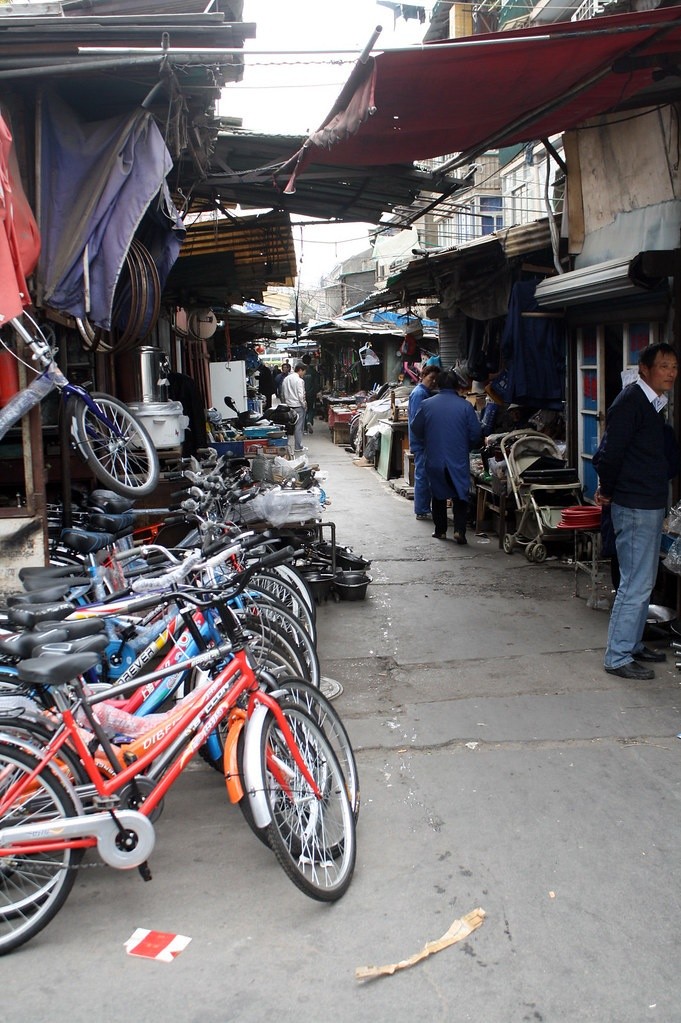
[606,660,655,680]
[633,647,666,662]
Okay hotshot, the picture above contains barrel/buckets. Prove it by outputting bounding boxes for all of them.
[132,345,168,402]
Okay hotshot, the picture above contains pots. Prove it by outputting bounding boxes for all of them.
[298,543,373,601]
[122,401,183,451]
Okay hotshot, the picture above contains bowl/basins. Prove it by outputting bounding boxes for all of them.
[267,431,286,439]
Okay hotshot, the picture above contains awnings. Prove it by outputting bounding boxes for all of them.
[180,206,298,288]
[181,125,477,233]
[285,5,681,197]
[342,212,549,322]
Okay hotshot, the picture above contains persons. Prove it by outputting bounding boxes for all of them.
[282,362,308,451]
[272,365,282,383]
[254,359,272,411]
[409,366,441,520]
[410,371,485,544]
[285,359,291,373]
[272,364,288,399]
[163,370,207,462]
[300,355,320,435]
[473,406,565,528]
[591,344,681,680]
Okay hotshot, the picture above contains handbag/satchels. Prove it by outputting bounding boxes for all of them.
[359,342,380,366]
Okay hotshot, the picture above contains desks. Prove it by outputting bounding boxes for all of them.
[322,395,356,420]
[377,419,408,482]
[474,484,514,550]
[329,405,351,444]
[117,445,183,475]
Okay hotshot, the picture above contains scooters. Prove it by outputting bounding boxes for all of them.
[224,396,299,436]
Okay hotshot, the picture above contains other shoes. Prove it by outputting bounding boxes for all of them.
[295,447,308,452]
[416,511,433,520]
[432,532,446,539]
[304,431,308,435]
[307,422,313,434]
[454,529,467,544]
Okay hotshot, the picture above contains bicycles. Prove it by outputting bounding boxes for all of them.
[0,308,361,956]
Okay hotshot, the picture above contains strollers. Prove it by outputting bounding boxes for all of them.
[481,429,592,563]
